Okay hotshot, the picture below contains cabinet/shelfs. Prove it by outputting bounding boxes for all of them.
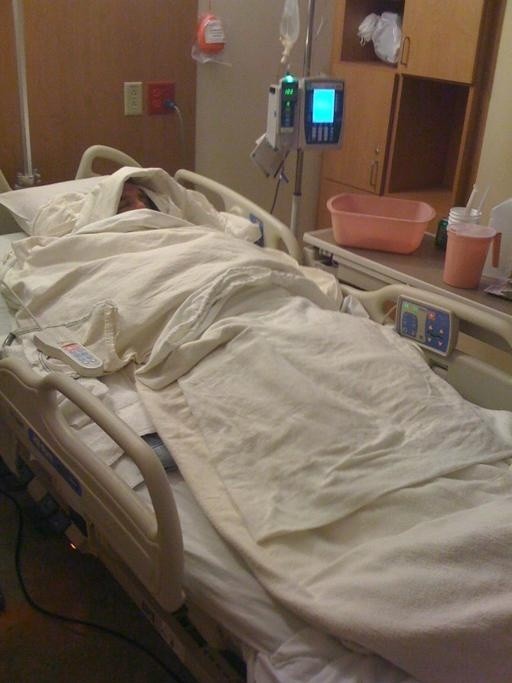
[312,1,508,232]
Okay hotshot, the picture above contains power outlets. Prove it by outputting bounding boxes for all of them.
[124,81,144,116]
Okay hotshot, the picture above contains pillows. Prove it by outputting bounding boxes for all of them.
[0,174,114,238]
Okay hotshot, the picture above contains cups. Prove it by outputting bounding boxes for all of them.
[442,207,502,288]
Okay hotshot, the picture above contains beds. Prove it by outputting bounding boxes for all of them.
[0,145,512,681]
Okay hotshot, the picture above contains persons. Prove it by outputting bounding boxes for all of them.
[118,184,159,214]
